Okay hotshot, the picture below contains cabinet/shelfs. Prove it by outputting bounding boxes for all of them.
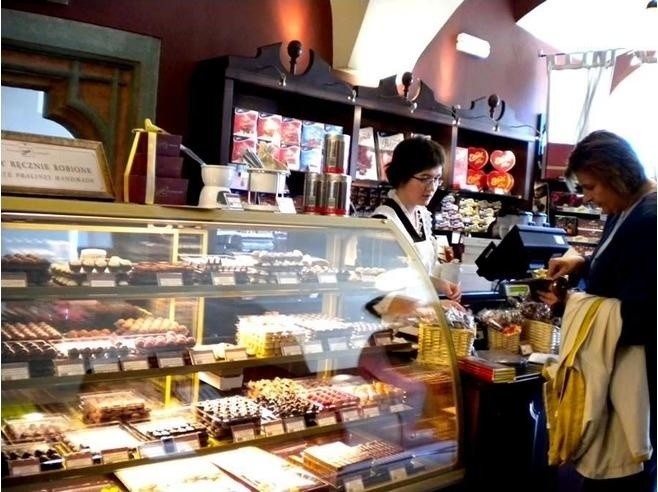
[181,37,543,331]
[2,193,465,492]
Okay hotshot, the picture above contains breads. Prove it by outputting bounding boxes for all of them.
[146,422,205,437]
[65,328,114,338]
[4,416,62,443]
[81,391,151,422]
[2,340,56,357]
[134,330,195,350]
[130,262,198,286]
[1,252,47,263]
[307,387,359,407]
[1,320,61,340]
[8,449,63,464]
[117,316,188,335]
[68,341,129,357]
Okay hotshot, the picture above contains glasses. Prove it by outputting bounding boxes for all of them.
[411,175,444,186]
[550,277,568,318]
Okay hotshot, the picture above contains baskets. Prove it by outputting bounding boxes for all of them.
[487,322,522,355]
[521,317,555,354]
[416,322,477,366]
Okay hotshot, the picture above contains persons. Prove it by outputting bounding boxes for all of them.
[334,136,462,326]
[533,128,656,490]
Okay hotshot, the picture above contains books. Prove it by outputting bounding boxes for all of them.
[287,438,413,488]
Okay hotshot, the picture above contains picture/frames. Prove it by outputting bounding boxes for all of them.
[0,128,117,202]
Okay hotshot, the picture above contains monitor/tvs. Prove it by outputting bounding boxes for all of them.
[476,223,569,280]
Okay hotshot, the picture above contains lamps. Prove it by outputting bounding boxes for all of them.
[253,65,543,146]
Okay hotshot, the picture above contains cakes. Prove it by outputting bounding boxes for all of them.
[235,311,311,358]
[49,247,132,288]
[356,380,405,404]
[288,307,352,342]
[197,393,261,438]
